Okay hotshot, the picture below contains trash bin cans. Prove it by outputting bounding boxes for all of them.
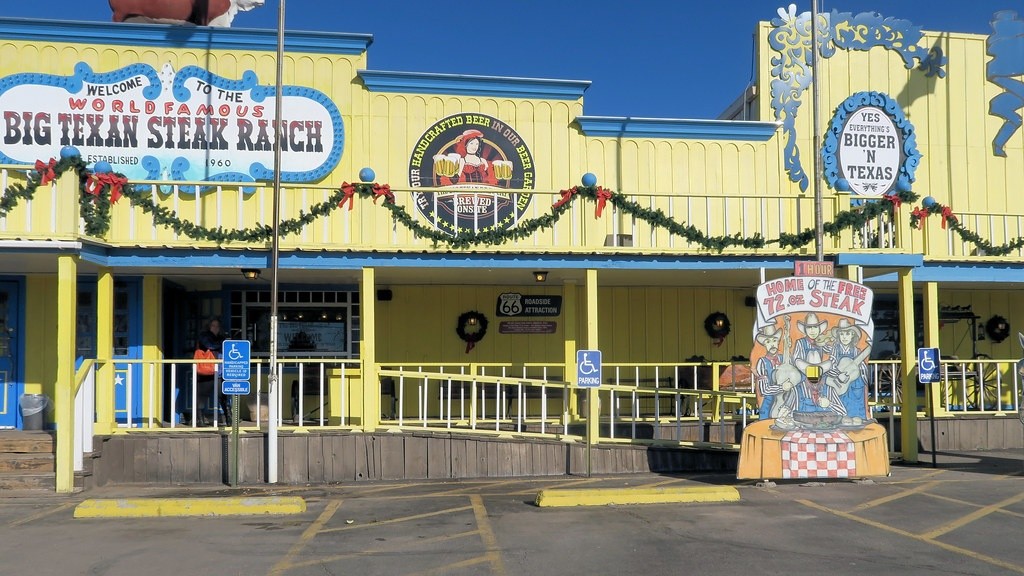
[22,393,45,429]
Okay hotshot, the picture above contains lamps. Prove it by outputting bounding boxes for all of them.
[978,322,986,340]
[998,321,1006,332]
[716,316,724,326]
[534,271,548,282]
[467,316,476,325]
[241,269,260,280]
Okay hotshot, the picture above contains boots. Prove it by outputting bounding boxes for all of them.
[222,405,243,426]
[197,408,206,427]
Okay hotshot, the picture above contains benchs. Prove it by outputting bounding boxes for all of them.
[286,376,397,422]
[606,377,686,416]
[438,378,578,419]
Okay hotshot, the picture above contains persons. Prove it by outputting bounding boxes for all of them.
[194,315,242,427]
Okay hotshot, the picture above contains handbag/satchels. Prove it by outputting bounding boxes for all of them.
[193,330,216,375]
